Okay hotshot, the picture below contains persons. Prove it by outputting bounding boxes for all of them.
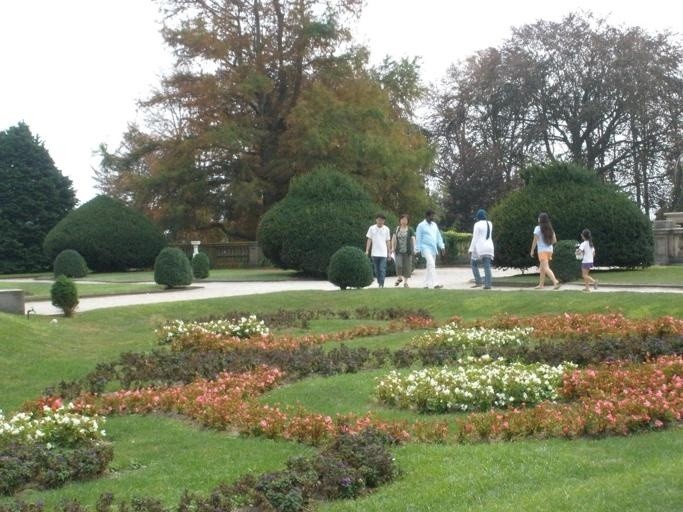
[467,210,495,289]
[530,213,560,289]
[391,214,415,287]
[575,228,598,291]
[363,214,391,287]
[416,211,446,288]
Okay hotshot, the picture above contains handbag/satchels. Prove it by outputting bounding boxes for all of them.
[391,234,397,252]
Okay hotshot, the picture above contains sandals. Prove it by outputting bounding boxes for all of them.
[395,278,403,286]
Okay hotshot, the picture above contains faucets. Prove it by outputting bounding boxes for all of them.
[27,307,36,319]
